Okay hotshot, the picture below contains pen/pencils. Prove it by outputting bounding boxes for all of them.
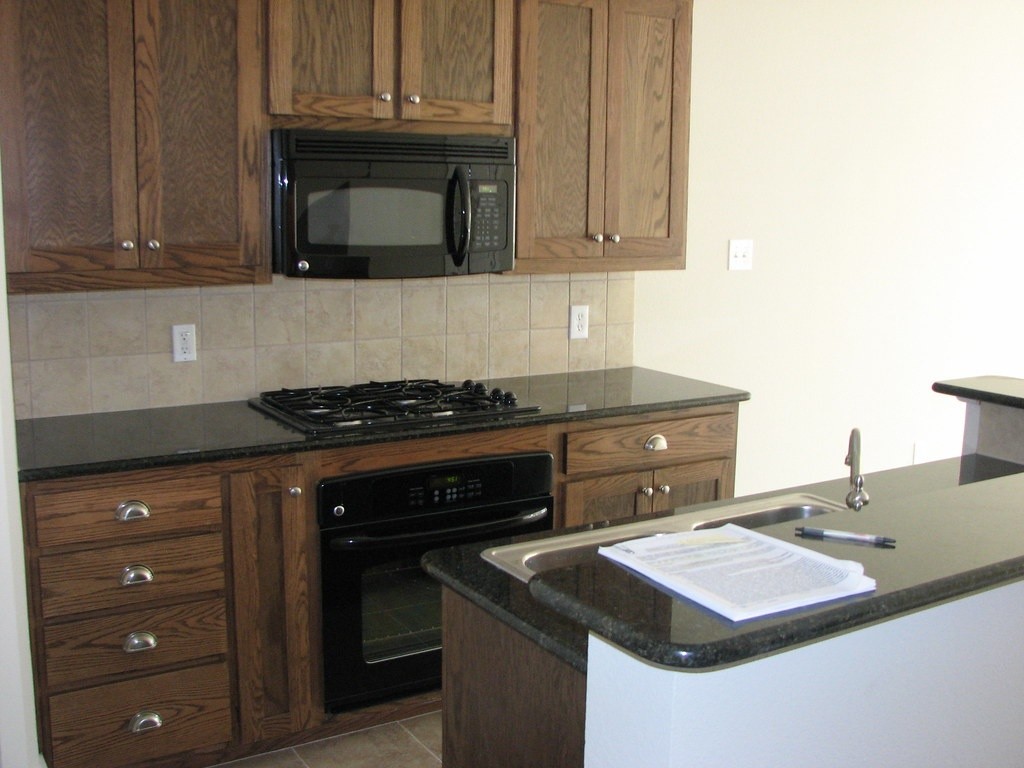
[795,526,896,545]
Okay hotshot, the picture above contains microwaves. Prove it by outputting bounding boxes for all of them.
[272,129,518,282]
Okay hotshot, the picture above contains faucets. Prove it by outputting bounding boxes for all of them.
[844,427,870,511]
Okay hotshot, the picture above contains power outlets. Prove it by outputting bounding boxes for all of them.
[569,304,589,339]
[726,238,755,271]
[171,324,197,363]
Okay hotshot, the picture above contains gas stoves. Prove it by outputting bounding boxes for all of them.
[247,378,540,436]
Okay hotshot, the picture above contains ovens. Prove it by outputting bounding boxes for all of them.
[316,451,556,715]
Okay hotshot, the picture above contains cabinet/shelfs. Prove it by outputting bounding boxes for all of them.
[441,585,585,768]
[19,450,316,767]
[0,0,272,294]
[267,0,517,138]
[553,399,741,532]
[489,0,696,277]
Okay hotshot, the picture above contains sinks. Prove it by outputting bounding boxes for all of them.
[480,515,678,584]
[686,492,848,530]
